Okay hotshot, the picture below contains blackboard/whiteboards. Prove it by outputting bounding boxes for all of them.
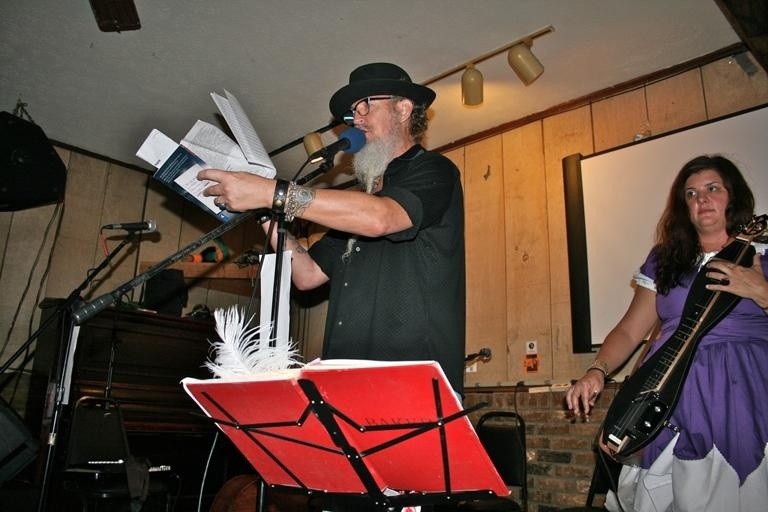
[562,104,768,352]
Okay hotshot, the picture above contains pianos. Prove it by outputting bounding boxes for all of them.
[23,297,258,512]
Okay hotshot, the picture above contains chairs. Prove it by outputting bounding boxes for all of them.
[475,411,529,512]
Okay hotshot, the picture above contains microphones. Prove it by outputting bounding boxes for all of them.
[311,126,365,162]
[104,219,157,232]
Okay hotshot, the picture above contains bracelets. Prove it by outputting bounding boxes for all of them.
[583,359,609,376]
[284,178,318,223]
[270,175,290,215]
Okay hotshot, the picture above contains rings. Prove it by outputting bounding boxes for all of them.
[221,203,226,210]
[719,274,730,286]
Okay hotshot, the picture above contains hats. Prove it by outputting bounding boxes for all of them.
[330,62,436,120]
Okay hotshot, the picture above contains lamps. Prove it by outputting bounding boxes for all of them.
[302,132,324,164]
[460,41,545,108]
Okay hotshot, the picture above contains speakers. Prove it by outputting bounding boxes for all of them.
[0,111,67,211]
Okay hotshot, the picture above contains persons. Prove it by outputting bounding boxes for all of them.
[563,151,767,511]
[196,61,471,412]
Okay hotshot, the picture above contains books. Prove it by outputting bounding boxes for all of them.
[177,353,512,502]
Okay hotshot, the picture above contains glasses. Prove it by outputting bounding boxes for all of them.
[344,97,391,125]
